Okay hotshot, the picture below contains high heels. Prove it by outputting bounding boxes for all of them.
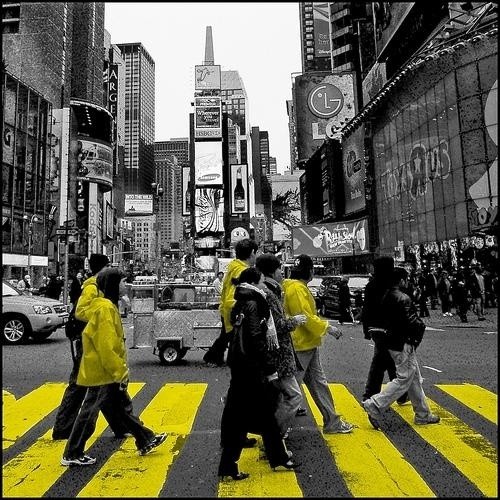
[271,456,301,471]
[220,471,249,484]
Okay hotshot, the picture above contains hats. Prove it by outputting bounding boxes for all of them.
[88,253,110,275]
[255,252,281,276]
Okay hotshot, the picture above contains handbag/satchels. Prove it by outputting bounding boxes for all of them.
[406,314,426,350]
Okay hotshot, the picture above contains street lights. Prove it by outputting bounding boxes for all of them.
[148,180,163,257]
[86,226,97,255]
[23,212,40,289]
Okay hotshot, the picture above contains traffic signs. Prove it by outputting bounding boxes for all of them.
[61,219,75,228]
[56,226,80,236]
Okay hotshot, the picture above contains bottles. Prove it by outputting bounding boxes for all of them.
[233,167,246,212]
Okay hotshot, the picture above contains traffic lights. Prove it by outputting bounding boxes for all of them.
[421,255,431,273]
[458,247,468,273]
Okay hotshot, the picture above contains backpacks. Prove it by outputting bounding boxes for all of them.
[64,282,99,342]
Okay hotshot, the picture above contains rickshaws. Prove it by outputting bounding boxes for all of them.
[130,283,222,366]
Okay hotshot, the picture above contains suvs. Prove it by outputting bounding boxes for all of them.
[319,274,373,321]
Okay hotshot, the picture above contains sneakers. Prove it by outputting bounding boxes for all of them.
[323,420,355,434]
[448,313,454,317]
[138,431,168,456]
[478,317,486,321]
[397,397,412,406]
[59,452,98,465]
[297,408,319,415]
[244,438,257,446]
[293,253,315,273]
[442,313,446,317]
[414,413,441,425]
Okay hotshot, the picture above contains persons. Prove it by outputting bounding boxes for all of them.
[280,253,355,435]
[337,258,498,325]
[221,253,307,458]
[219,238,307,417]
[16,271,93,303]
[361,267,441,431]
[52,253,144,440]
[218,267,302,483]
[61,267,168,467]
[362,256,412,404]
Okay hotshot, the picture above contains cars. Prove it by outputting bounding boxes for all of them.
[3,280,69,344]
[306,275,326,308]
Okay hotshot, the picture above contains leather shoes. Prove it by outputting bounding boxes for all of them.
[361,399,382,431]
[202,357,227,367]
